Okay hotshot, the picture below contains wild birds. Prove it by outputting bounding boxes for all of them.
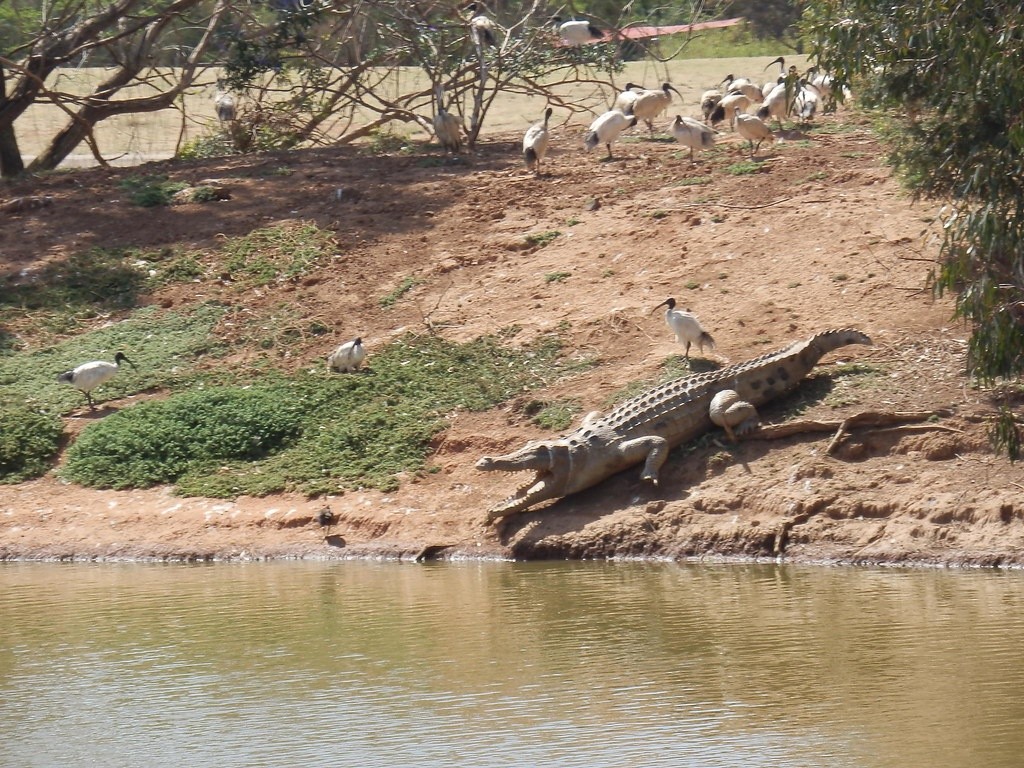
[732,106,774,156]
[615,55,852,140]
[212,76,236,130]
[522,106,552,175]
[653,297,714,364]
[58,352,139,414]
[326,336,364,372]
[548,15,605,54]
[669,114,718,162]
[583,110,638,158]
[462,2,498,48]
